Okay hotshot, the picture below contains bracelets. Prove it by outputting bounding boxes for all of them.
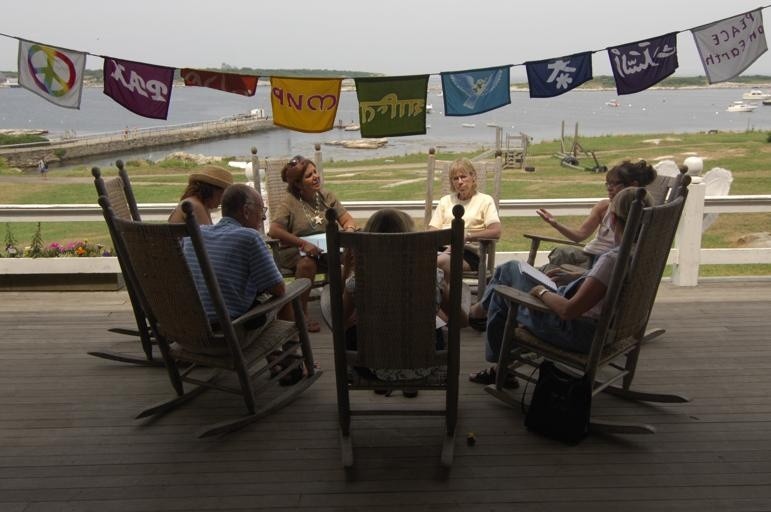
[466,231,472,241]
[538,288,550,299]
[297,240,305,251]
[342,224,355,231]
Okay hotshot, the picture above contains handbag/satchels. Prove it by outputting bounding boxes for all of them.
[524,360,596,447]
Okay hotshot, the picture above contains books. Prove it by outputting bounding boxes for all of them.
[517,260,557,293]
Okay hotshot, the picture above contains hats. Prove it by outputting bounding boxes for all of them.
[188,166,234,188]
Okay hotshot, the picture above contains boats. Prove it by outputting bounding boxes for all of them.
[737,89,771,106]
[724,99,759,117]
[335,118,364,133]
[460,123,476,130]
[421,100,434,115]
[601,97,622,111]
[485,122,502,128]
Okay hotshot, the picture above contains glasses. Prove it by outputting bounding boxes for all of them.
[288,155,303,167]
[605,182,624,187]
[248,201,268,215]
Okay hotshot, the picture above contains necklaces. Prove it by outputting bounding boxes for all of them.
[299,188,323,231]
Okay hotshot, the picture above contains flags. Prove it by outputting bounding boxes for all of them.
[15,37,85,111]
[267,74,342,134]
[352,72,429,139]
[99,54,175,121]
[605,31,680,96]
[439,63,511,116]
[524,49,594,98]
[178,66,259,98]
[688,6,768,85]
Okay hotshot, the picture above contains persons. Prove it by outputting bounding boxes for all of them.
[178,181,324,386]
[424,158,502,288]
[461,186,655,389]
[532,156,658,288]
[167,165,235,231]
[265,154,355,333]
[340,206,468,399]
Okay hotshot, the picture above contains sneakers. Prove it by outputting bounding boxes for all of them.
[469,367,519,388]
[305,320,321,331]
[469,311,488,331]
